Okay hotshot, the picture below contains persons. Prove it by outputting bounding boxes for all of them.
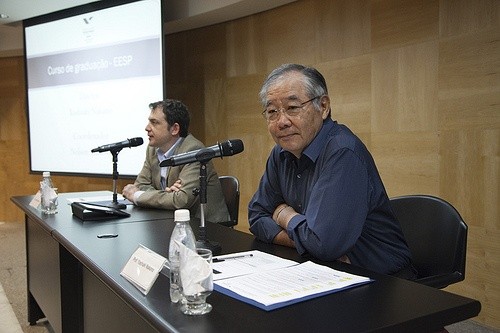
[122,99,233,230]
[247,63,420,288]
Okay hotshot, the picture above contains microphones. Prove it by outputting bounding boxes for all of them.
[159,139,244,168]
[91,137,144,153]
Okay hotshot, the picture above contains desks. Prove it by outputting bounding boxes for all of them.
[11,190,483,333]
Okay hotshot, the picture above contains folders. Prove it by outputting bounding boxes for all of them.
[206,249,375,312]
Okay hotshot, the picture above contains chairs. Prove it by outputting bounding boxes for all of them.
[390,195,469,333]
[218,176,240,229]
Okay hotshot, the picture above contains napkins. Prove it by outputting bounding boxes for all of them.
[40,182,57,204]
[173,239,214,296]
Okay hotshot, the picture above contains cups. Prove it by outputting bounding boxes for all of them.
[179,248,213,315]
[44,188,58,215]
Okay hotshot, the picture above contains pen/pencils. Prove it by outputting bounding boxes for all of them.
[212,253,253,262]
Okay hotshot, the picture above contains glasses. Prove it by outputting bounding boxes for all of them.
[261,94,324,121]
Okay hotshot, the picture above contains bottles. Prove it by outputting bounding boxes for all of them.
[40,172,54,211]
[168,209,197,305]
